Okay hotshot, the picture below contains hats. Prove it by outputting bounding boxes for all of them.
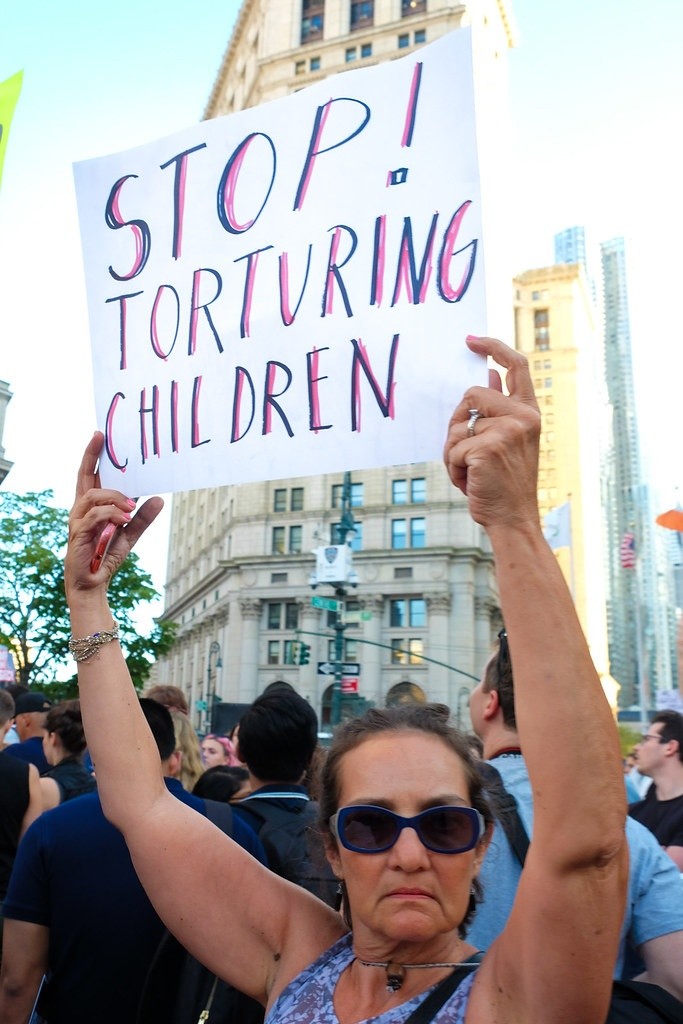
[10,693,51,719]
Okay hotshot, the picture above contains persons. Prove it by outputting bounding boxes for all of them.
[0,627,683,1024]
[65,334,630,1024]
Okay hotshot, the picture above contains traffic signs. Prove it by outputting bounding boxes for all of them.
[317,661,361,676]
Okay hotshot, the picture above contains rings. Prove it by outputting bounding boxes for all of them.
[468,409,485,438]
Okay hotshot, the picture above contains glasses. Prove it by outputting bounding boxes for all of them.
[329,804,485,855]
[640,733,666,745]
[497,629,507,684]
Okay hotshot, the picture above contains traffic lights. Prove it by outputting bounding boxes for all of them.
[300,642,312,665]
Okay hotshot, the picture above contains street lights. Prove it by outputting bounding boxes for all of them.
[206,640,224,735]
[323,470,360,727]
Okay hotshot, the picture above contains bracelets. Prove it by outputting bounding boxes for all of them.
[69,618,120,665]
